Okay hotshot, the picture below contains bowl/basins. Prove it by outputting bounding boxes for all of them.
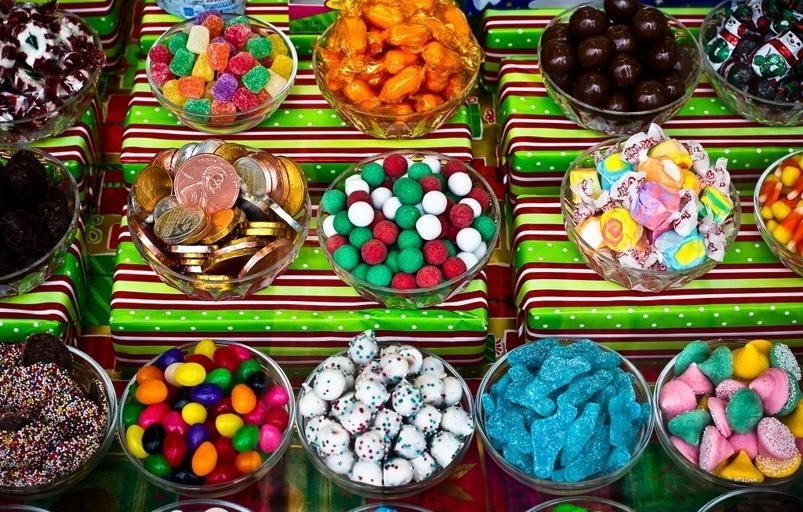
[294,343,476,500]
[697,0,803,127]
[346,500,433,511]
[0,343,119,497]
[114,339,296,496]
[123,146,310,299]
[311,20,480,138]
[315,152,501,313]
[524,495,633,512]
[691,489,801,511]
[754,149,802,277]
[536,0,704,137]
[558,139,743,297]
[652,335,801,487]
[473,337,657,494]
[0,141,78,300]
[0,11,103,147]
[150,496,250,512]
[144,11,300,135]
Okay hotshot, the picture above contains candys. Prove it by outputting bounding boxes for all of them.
[702,2,803,124]
[537,2,700,134]
[753,150,802,279]
[1,334,117,501]
[657,339,803,488]
[299,329,476,497]
[130,137,309,300]
[144,10,297,132]
[1,148,80,296]
[560,122,744,294]
[0,8,106,142]
[316,151,503,308]
[312,0,482,138]
[480,337,655,495]
[118,330,295,494]
